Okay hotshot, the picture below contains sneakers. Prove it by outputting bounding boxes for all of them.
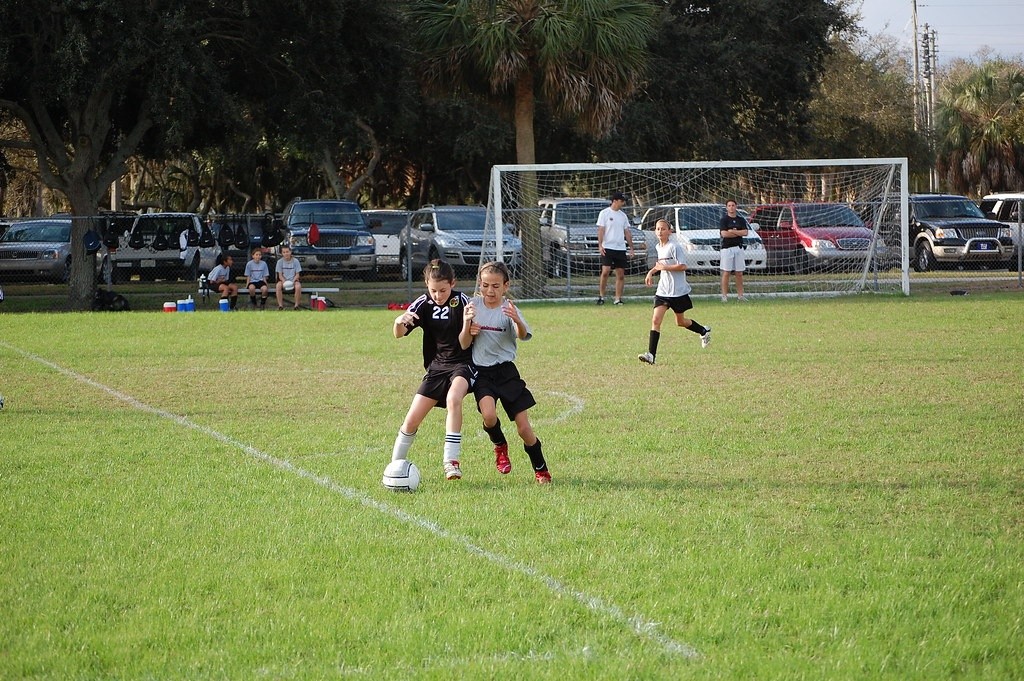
[614,300,625,305]
[597,296,604,304]
[279,306,283,311]
[444,460,462,480]
[535,470,551,484]
[721,296,728,302]
[700,326,712,348]
[737,296,749,302]
[638,352,656,365]
[294,306,299,310]
[494,442,511,474]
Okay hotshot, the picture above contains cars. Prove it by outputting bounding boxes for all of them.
[636,203,768,275]
[88,211,285,283]
[0,219,112,285]
[978,193,1024,271]
[360,208,409,282]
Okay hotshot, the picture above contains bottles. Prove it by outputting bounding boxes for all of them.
[199,274,205,289]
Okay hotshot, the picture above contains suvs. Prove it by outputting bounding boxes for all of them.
[747,200,887,275]
[399,203,522,281]
[515,196,648,278]
[270,196,377,282]
[858,192,1014,273]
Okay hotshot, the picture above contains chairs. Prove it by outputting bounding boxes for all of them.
[60,228,69,240]
[949,202,967,214]
[918,203,936,215]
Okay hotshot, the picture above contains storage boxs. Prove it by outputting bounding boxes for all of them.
[310,294,318,310]
[163,302,177,312]
[177,299,186,311]
[220,299,230,311]
[317,297,327,311]
[186,299,195,312]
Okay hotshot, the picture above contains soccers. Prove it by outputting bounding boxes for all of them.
[383,458,420,491]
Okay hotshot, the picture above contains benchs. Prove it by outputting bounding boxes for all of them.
[228,288,340,311]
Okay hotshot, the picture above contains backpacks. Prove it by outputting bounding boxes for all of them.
[307,213,319,246]
[103,217,119,249]
[128,217,145,250]
[84,216,102,252]
[262,214,285,247]
[152,214,250,251]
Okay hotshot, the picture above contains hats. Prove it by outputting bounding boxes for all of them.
[613,193,628,201]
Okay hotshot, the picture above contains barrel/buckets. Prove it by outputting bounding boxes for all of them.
[163,295,195,311]
[219,299,228,311]
[311,295,326,311]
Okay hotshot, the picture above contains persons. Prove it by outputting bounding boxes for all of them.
[638,220,711,365]
[275,245,302,311]
[391,260,478,479]
[596,193,634,305]
[459,262,552,483]
[719,200,750,303]
[245,248,269,311]
[207,253,239,312]
